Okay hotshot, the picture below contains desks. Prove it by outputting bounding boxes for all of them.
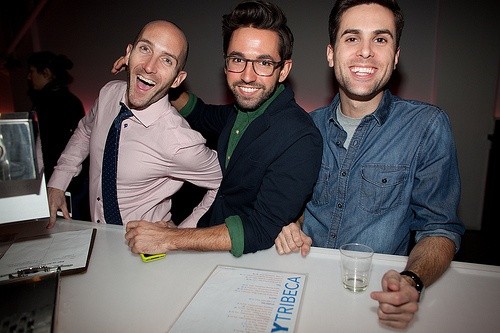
[0,219,500,333]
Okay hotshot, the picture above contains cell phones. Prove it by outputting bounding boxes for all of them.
[57,191,73,218]
[140,254,166,262]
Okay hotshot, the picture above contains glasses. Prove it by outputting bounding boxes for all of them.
[225,55,285,77]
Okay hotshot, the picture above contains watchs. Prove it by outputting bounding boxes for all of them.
[400,270,427,303]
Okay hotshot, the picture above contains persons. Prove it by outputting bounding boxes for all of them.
[275,0,461,328]
[47,18,223,229]
[19,53,89,221]
[111,0,324,258]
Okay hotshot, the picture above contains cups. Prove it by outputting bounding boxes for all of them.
[338,243,374,292]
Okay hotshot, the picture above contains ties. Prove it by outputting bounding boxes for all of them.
[102,102,134,225]
